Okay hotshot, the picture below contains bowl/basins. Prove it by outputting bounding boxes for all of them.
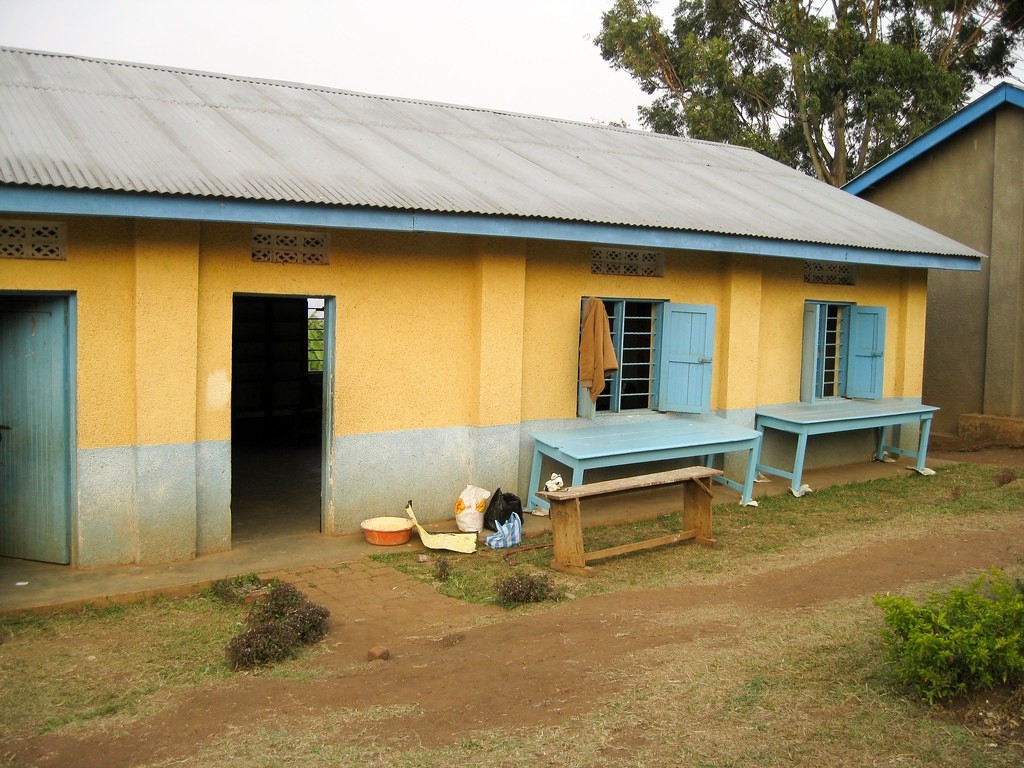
[360,517,416,546]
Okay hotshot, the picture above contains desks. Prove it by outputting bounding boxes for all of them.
[754,397,941,494]
[529,413,763,513]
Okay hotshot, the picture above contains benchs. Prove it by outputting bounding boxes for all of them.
[535,466,727,578]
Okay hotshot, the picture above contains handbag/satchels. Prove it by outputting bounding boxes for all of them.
[483,487,524,533]
[455,483,492,533]
[485,512,522,549]
